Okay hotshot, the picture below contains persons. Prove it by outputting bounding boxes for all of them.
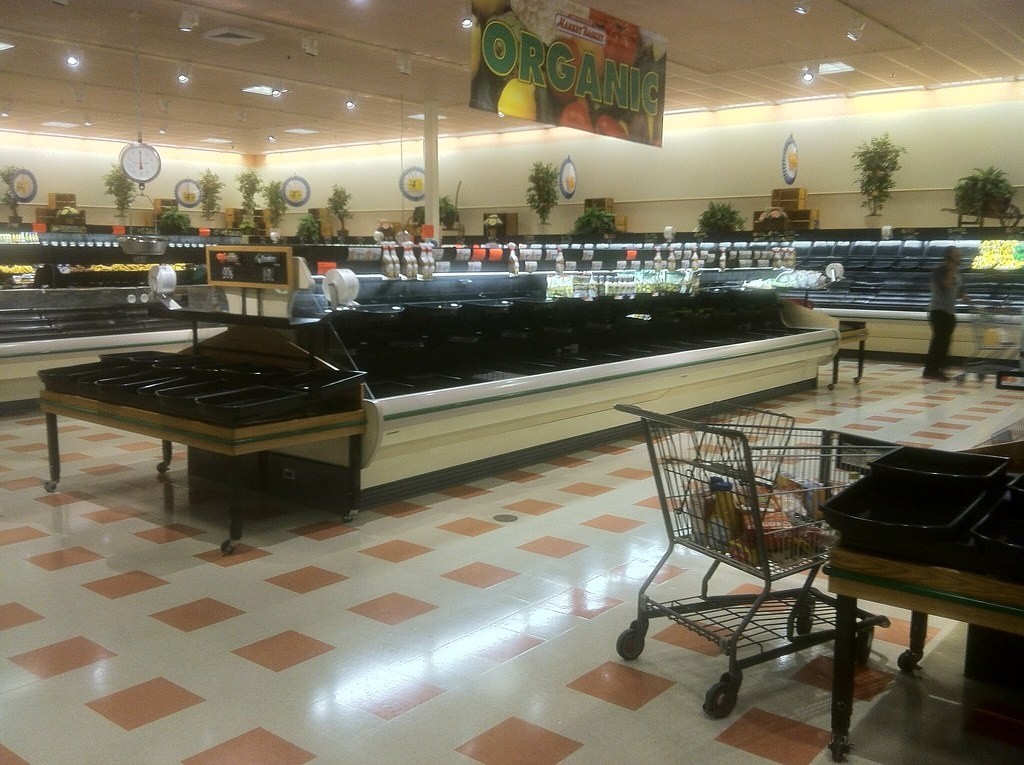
[921,245,965,383]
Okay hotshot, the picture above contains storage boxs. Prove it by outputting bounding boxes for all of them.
[35,349,370,427]
[332,284,818,402]
[816,442,1024,584]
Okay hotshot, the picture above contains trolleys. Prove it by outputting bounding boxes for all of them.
[613,397,891,720]
[955,287,1023,388]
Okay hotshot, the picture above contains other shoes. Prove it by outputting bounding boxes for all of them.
[923,369,949,381]
